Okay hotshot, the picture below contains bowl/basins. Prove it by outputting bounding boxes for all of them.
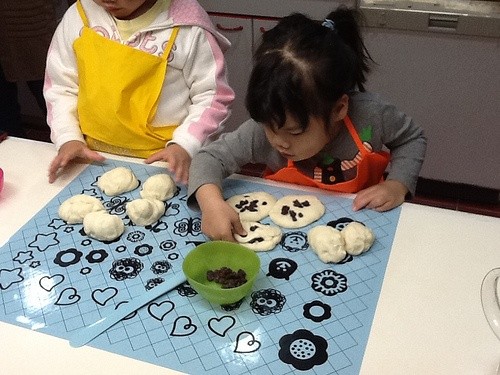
[183,240,260,305]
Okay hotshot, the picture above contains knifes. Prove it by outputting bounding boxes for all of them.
[70,271,187,347]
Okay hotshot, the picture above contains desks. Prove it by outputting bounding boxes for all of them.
[1,136,500,375]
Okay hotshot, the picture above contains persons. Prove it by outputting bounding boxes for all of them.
[186,6,429,247]
[42,0,237,186]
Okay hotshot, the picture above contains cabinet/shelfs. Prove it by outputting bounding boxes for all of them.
[191,0,500,195]
